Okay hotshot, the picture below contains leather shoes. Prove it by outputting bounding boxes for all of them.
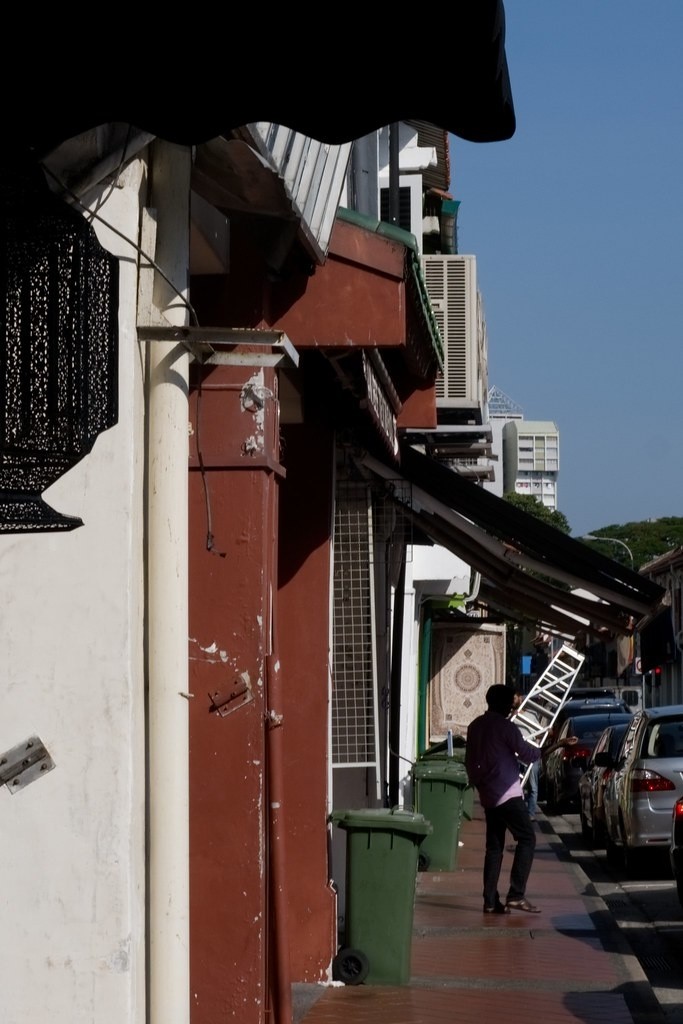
[508,899,541,914]
[483,899,510,914]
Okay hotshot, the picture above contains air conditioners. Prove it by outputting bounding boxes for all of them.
[422,252,493,427]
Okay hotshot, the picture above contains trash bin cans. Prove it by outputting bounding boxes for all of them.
[412,735,474,871]
[329,808,433,986]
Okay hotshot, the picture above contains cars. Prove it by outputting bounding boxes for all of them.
[526,685,683,900]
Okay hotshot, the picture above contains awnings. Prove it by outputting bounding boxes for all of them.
[1,0,515,165]
[348,435,665,644]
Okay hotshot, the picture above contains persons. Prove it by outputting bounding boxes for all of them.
[506,691,542,821]
[466,684,578,915]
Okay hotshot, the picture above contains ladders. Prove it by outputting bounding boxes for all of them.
[508,642,585,788]
